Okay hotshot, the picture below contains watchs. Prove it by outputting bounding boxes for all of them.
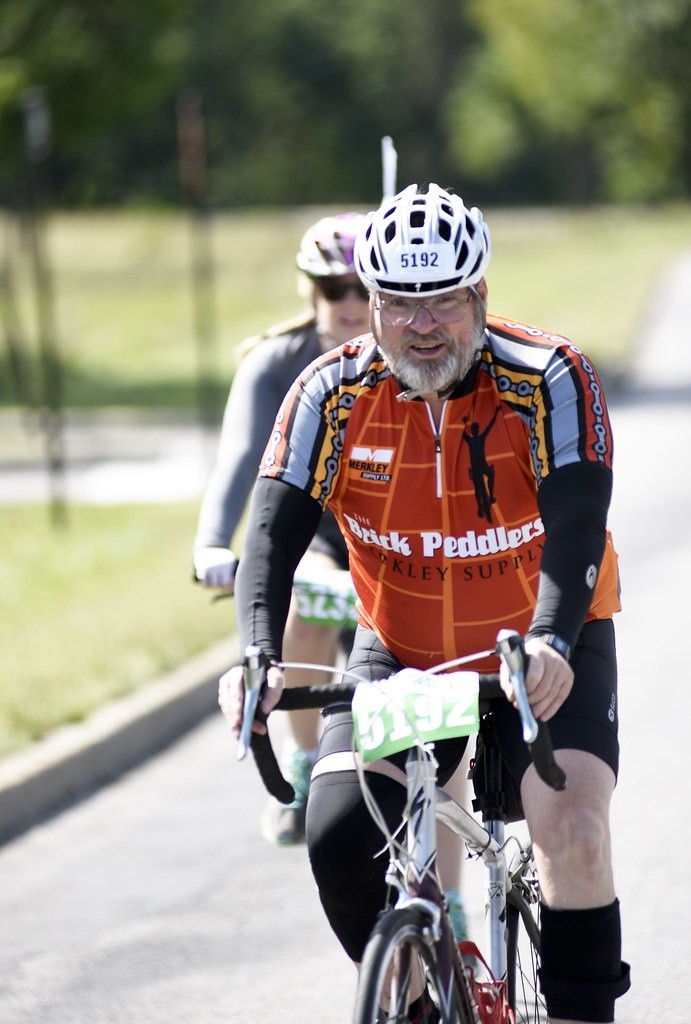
[540,633,570,662]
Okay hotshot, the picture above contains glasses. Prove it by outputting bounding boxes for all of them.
[314,278,369,300]
[372,290,474,326]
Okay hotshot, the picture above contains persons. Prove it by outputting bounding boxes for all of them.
[194,213,482,982]
[214,184,631,1024]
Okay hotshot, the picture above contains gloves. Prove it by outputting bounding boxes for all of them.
[196,547,239,589]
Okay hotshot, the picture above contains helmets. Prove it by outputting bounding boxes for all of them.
[354,183,491,297]
[296,211,364,276]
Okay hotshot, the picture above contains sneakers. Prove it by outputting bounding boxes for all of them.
[447,890,480,980]
[263,739,319,844]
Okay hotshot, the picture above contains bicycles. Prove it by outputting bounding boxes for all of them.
[192,559,552,1021]
